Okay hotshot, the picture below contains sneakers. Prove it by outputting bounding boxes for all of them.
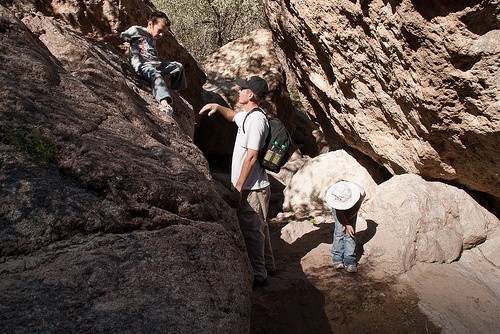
[332,259,345,269]
[345,264,357,272]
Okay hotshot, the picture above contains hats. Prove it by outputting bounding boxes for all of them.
[325,181,361,210]
[236,76,268,97]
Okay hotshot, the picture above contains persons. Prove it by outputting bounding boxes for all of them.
[118,10,187,116]
[326,180,366,272]
[199,76,278,291]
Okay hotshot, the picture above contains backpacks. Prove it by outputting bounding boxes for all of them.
[242,108,292,173]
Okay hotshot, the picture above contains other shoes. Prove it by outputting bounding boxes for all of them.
[253,277,270,286]
[268,269,277,275]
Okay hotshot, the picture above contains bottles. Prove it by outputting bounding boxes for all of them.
[263,140,279,166]
[268,144,286,170]
